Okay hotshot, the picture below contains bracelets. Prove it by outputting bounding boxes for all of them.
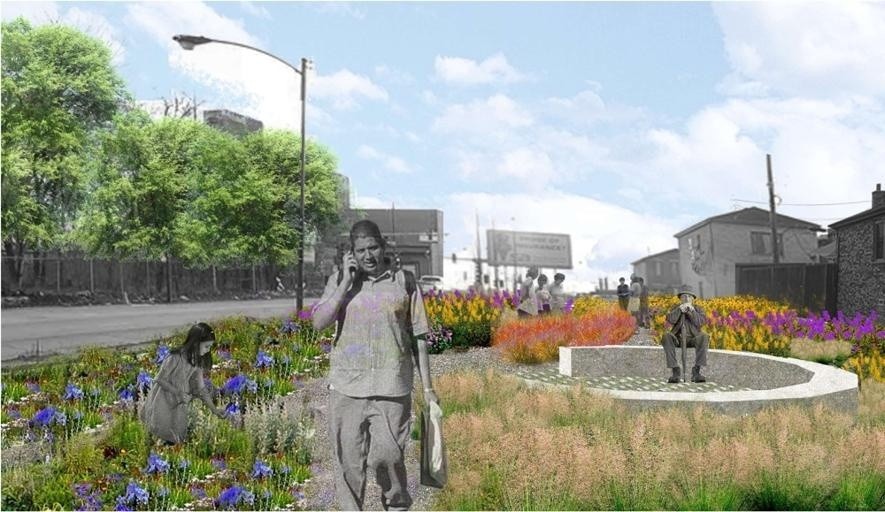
[422,387,433,393]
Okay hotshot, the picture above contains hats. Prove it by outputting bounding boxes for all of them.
[677,284,697,300]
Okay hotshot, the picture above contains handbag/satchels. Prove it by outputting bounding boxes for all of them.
[420,399,447,490]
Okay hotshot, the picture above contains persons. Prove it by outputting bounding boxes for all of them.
[639,276,650,314]
[516,266,539,318]
[134,321,230,444]
[617,277,630,311]
[626,274,642,314]
[535,274,552,314]
[661,283,711,383]
[308,219,450,511]
[550,272,566,309]
